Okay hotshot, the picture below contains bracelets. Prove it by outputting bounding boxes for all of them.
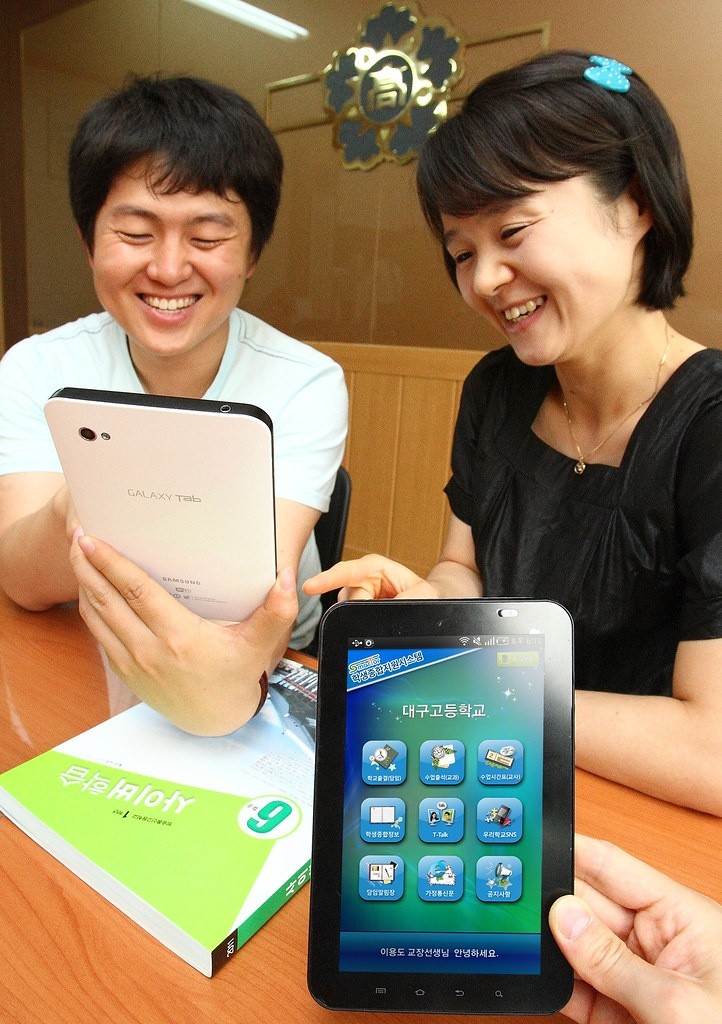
[253,671,269,718]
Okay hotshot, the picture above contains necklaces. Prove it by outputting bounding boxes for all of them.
[561,319,671,475]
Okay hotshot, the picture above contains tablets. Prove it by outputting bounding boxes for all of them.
[308,596,574,1016]
[45,387,276,624]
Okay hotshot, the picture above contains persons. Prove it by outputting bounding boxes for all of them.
[547,832,722,1024]
[0,73,347,738]
[301,48,722,824]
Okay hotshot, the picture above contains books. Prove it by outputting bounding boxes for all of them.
[0,658,319,977]
